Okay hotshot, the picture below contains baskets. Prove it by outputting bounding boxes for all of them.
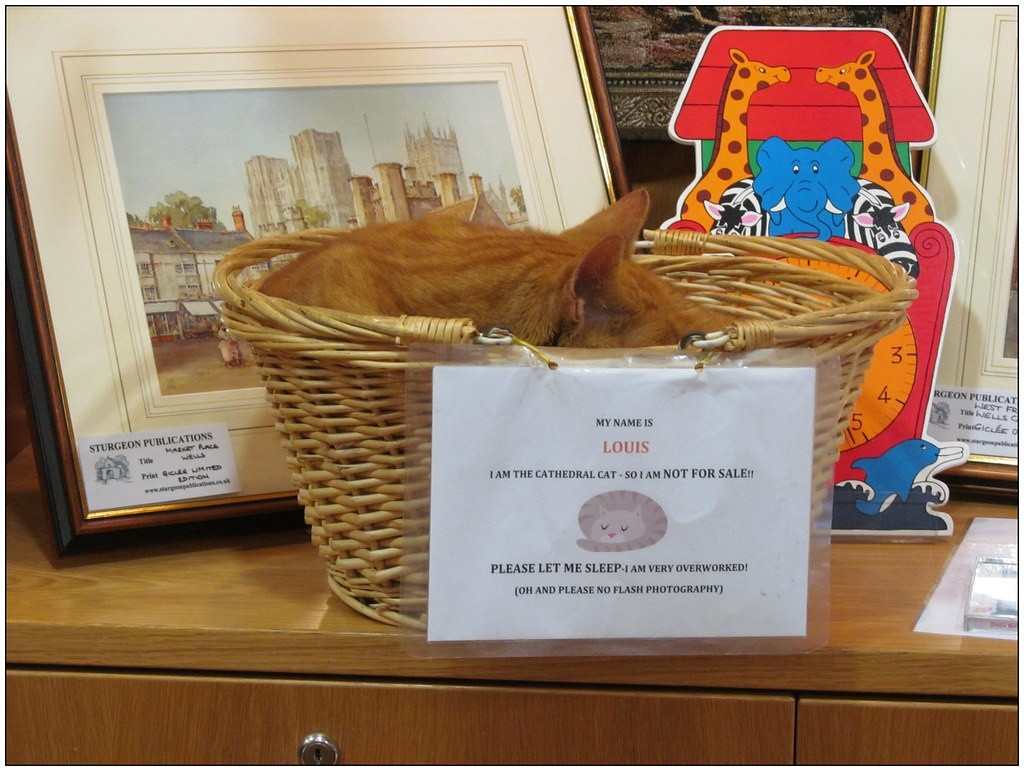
[211,228,920,631]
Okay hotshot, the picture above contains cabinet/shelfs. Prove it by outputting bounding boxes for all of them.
[6,437,1018,767]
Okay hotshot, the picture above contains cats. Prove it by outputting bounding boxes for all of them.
[260,187,749,347]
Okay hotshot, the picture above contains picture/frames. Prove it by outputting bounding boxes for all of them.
[906,5,1018,505]
[6,6,651,565]
